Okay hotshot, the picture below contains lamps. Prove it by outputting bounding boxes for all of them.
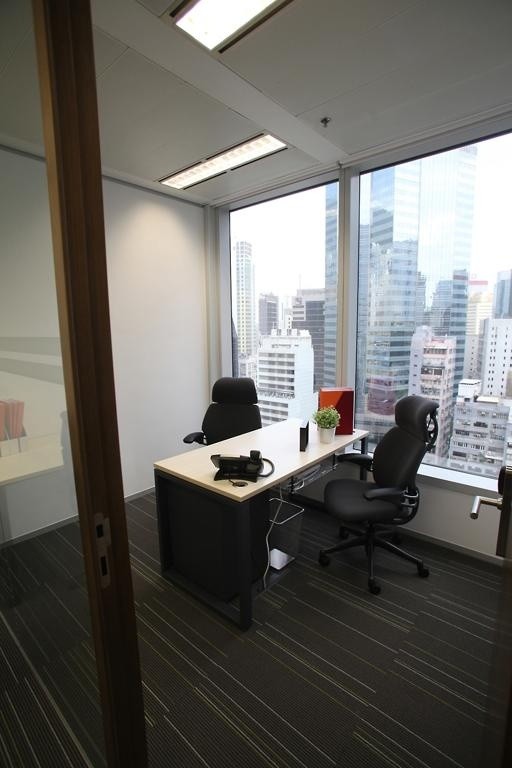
[170,0,293,54]
[154,129,287,190]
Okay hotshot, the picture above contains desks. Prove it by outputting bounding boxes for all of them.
[155,417,370,632]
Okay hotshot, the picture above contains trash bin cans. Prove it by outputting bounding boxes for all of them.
[267,498,304,571]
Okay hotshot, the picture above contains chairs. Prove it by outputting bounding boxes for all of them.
[183,377,262,445]
[318,394,440,595]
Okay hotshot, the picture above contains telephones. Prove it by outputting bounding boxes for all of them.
[211,450,263,482]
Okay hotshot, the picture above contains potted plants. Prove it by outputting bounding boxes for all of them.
[313,407,341,444]
